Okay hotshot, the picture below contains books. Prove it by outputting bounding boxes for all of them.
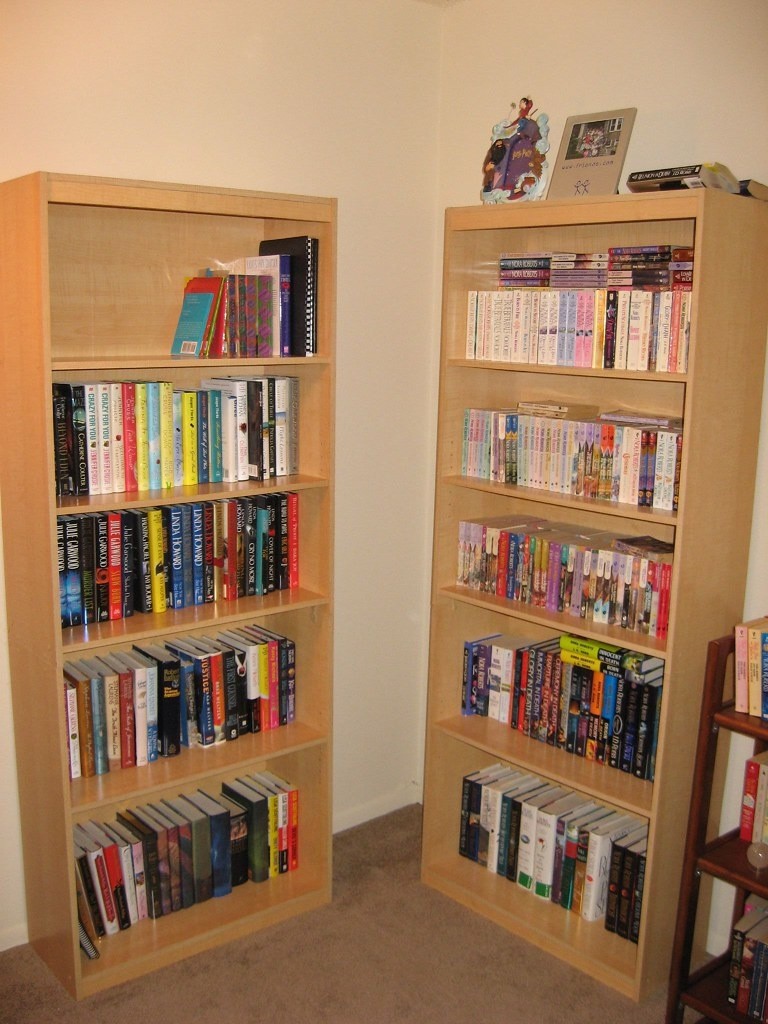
[625,160,768,202]
[51,231,318,963]
[456,247,767,1023]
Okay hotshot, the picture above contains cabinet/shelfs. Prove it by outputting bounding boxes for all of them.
[420,186,768,1001]
[0,173,338,1001]
[666,636,768,1024]
